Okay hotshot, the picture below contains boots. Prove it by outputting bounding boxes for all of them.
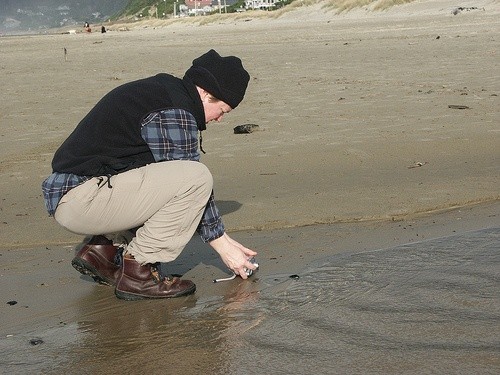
[115,252,196,301]
[72,234,123,286]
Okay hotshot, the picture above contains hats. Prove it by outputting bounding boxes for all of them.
[185,49,250,109]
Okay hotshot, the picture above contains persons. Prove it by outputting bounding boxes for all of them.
[101,26,106,33]
[84,22,89,27]
[42,49,258,301]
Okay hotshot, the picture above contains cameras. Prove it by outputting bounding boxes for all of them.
[244,256,256,277]
